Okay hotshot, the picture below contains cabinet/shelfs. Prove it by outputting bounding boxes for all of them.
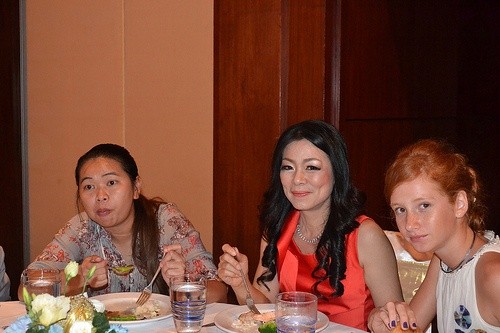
[212,0,500,307]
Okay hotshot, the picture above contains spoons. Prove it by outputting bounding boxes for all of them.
[93,260,133,275]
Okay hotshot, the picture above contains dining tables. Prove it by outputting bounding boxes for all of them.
[0,301,367,333]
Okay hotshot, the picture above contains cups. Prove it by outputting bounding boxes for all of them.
[276,292,318,333]
[169,274,206,333]
[21,268,60,312]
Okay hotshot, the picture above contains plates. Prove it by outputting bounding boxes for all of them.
[213,304,329,333]
[89,292,173,325]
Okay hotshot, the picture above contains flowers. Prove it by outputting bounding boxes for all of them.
[21,260,115,333]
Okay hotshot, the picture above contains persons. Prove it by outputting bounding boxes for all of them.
[367,140,500,333]
[217,119,405,333]
[18,143,228,304]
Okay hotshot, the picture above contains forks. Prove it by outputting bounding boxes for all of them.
[135,251,167,306]
[233,248,261,315]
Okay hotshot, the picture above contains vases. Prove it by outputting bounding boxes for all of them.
[4,314,128,333]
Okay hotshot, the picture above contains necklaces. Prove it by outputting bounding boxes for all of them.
[440,230,475,273]
[297,221,324,244]
